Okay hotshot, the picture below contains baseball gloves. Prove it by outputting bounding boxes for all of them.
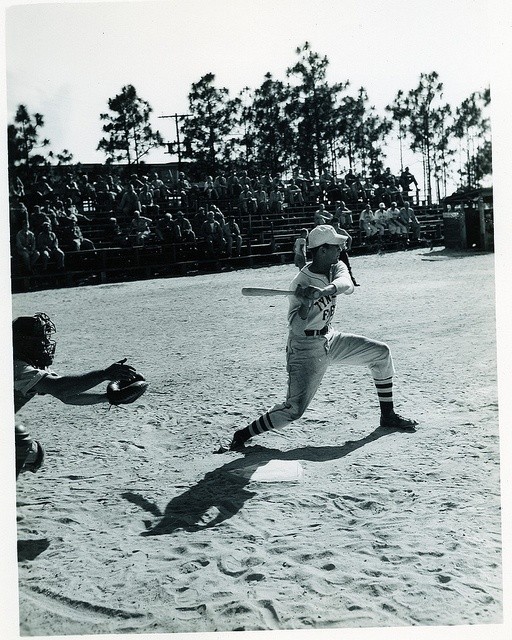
[106,373,148,410]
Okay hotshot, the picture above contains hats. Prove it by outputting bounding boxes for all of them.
[306,225,348,248]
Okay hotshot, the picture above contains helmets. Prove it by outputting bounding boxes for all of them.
[12,311,56,366]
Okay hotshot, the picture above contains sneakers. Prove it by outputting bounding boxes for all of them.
[380,412,418,428]
[230,429,248,450]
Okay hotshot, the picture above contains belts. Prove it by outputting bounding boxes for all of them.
[305,327,329,336]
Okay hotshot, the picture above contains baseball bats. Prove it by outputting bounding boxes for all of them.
[241,287,319,299]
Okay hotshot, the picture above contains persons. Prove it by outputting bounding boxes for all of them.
[386,179,403,201]
[119,184,140,216]
[92,175,104,185]
[209,205,225,229]
[151,172,165,189]
[16,221,41,264]
[40,200,59,226]
[287,180,304,205]
[293,227,309,271]
[52,200,68,217]
[374,180,392,206]
[78,175,96,192]
[37,222,65,271]
[226,216,243,259]
[350,177,367,198]
[200,211,226,257]
[273,172,284,188]
[13,311,137,481]
[140,185,153,212]
[215,171,228,194]
[31,176,60,200]
[8,176,26,196]
[64,173,80,191]
[129,174,144,191]
[331,217,361,287]
[9,195,29,229]
[106,176,122,193]
[314,203,338,227]
[251,175,260,183]
[63,214,96,251]
[176,171,192,208]
[229,223,420,448]
[399,167,420,191]
[269,186,285,219]
[289,172,304,186]
[244,177,250,184]
[399,201,424,242]
[203,176,220,200]
[318,168,334,182]
[304,171,313,181]
[129,210,153,246]
[260,172,273,184]
[339,179,351,191]
[387,202,410,245]
[345,169,356,185]
[104,217,121,240]
[239,170,251,185]
[98,183,117,201]
[29,205,51,224]
[195,207,207,226]
[371,169,382,185]
[375,202,396,243]
[79,174,96,195]
[141,176,152,190]
[157,212,174,237]
[335,201,355,229]
[382,167,396,179]
[359,204,379,246]
[156,184,173,208]
[253,185,269,220]
[174,210,197,250]
[363,178,376,198]
[227,170,244,195]
[64,198,94,223]
[239,185,258,213]
